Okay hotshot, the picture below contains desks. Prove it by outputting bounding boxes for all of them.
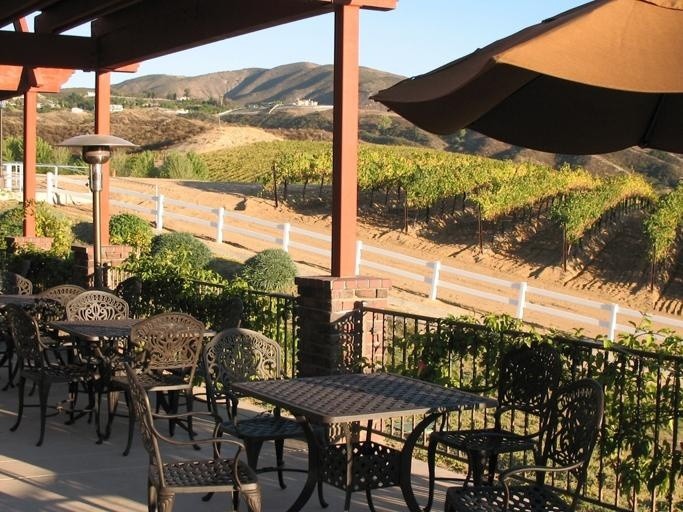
[230,370,499,511]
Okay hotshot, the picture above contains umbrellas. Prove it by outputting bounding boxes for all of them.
[366,0,683,156]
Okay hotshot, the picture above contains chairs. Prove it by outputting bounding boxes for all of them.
[444,383,604,510]
[424,345,562,511]
[202,328,328,508]
[0,271,223,458]
[123,363,264,512]
